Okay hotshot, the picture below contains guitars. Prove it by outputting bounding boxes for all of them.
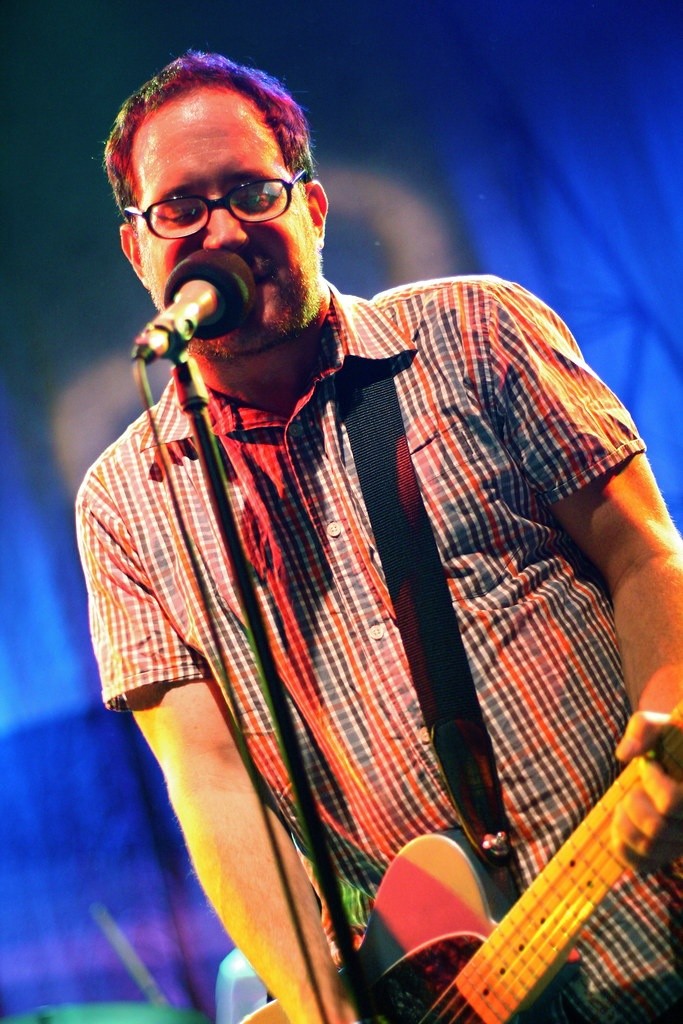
[243,702,682,1023]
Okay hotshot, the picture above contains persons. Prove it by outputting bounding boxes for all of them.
[74,50,683,1024]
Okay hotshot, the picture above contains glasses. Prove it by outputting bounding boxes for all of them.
[124,167,308,241]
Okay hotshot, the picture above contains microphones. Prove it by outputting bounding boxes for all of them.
[127,245,258,363]
